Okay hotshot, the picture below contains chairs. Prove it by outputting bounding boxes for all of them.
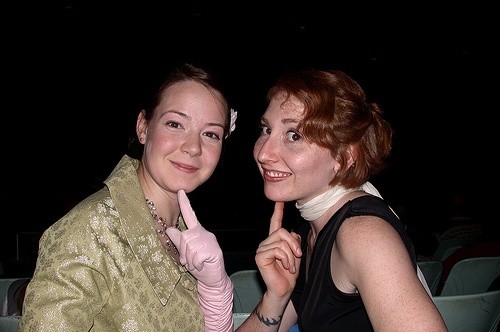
[7,279,31,316]
[230,270,267,313]
[418,255,500,332]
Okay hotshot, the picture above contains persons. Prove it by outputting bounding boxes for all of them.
[416,193,481,262]
[232,71,451,332]
[18,62,234,332]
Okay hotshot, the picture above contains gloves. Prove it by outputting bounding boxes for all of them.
[167,190,234,331]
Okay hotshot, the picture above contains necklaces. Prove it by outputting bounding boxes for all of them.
[145,196,182,257]
